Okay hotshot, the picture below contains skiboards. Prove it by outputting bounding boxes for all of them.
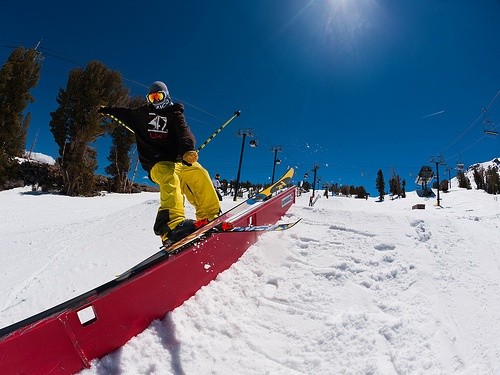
[115,167,302,277]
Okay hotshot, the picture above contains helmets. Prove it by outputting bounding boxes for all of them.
[146,81,171,109]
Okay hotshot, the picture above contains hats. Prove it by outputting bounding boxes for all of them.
[216,174,220,176]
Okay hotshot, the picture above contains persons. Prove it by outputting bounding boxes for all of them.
[98,81,222,247]
[212,174,222,201]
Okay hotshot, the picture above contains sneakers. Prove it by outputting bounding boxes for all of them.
[161,218,209,255]
[204,221,233,236]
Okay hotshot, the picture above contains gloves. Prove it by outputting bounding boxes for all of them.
[183,150,198,167]
